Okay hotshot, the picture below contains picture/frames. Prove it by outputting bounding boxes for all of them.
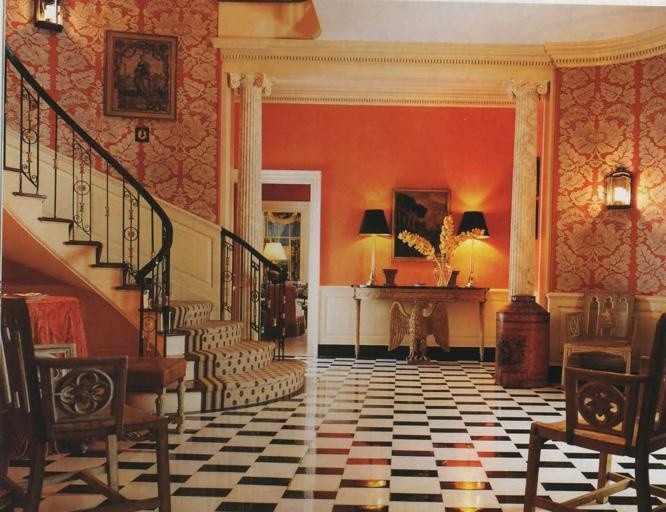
[104,30,178,121]
[391,188,450,261]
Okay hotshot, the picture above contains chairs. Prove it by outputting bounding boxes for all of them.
[561,287,640,392]
[523,313,666,512]
[0,295,172,511]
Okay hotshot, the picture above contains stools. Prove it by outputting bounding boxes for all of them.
[128,358,187,441]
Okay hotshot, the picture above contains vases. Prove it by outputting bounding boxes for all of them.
[433,260,452,287]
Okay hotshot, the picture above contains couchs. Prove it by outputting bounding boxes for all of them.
[264,284,305,337]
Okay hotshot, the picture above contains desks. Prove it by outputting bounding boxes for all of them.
[351,284,490,361]
[26,296,88,358]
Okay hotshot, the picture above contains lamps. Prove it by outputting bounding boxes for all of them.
[605,167,632,208]
[33,0,64,32]
[357,209,391,286]
[264,243,288,260]
[456,211,490,287]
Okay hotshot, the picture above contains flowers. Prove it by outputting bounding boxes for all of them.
[398,215,485,286]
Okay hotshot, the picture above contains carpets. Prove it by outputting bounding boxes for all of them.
[167,300,305,413]
